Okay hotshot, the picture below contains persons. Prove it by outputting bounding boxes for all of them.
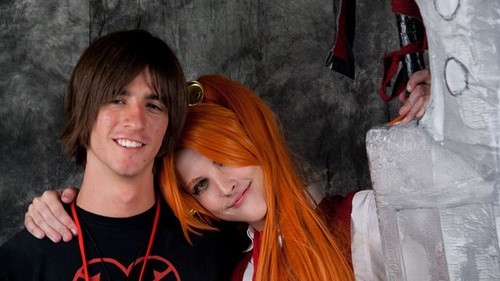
[0,29,251,281]
[24,69,432,281]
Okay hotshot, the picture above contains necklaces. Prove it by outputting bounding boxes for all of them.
[70,193,162,281]
